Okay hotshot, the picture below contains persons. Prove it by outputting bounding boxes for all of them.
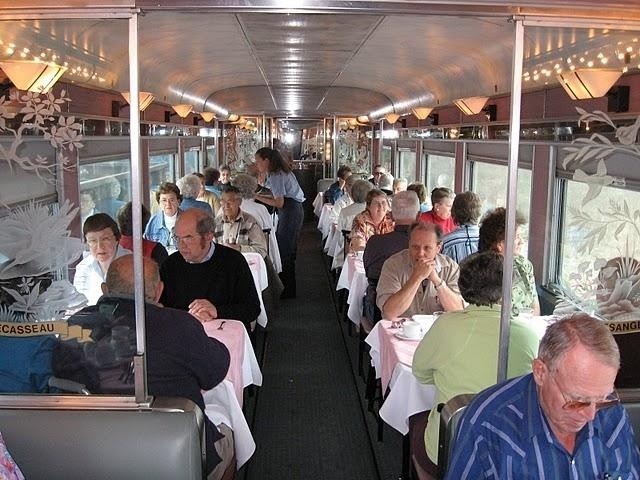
[94,177,129,224]
[161,209,261,334]
[118,202,169,260]
[362,191,421,326]
[53,253,234,480]
[312,151,317,160]
[324,165,353,205]
[218,164,233,187]
[310,147,313,159]
[202,166,224,199]
[376,174,395,195]
[63,212,134,322]
[245,163,276,215]
[416,185,460,238]
[213,186,269,260]
[337,179,389,249]
[350,190,396,251]
[330,175,364,225]
[444,314,640,480]
[142,182,188,252]
[248,162,260,179]
[78,191,98,232]
[193,173,221,218]
[410,250,541,480]
[254,147,306,296]
[176,175,214,217]
[407,183,434,214]
[439,193,481,260]
[367,164,386,186]
[478,208,539,314]
[213,173,283,275]
[0,258,55,396]
[386,179,407,213]
[375,222,469,322]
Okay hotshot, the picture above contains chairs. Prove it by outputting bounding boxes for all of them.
[316,176,339,193]
[438,387,640,480]
[0,395,206,480]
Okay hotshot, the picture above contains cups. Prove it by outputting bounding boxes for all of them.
[432,311,444,321]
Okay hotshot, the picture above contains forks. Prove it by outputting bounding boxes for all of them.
[217,321,228,330]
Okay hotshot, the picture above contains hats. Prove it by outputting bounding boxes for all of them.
[380,174,395,191]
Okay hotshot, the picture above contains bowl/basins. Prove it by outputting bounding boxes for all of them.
[403,321,422,337]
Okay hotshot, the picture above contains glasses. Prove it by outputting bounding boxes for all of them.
[373,170,384,176]
[171,235,201,244]
[552,375,620,411]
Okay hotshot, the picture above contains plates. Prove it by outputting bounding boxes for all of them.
[412,315,436,326]
[394,331,425,340]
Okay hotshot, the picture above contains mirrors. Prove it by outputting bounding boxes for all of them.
[278,119,325,162]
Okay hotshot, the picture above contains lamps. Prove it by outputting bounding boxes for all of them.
[557,68,624,100]
[0,61,66,94]
[118,92,155,111]
[201,112,214,122]
[171,105,194,119]
[386,116,399,123]
[412,107,432,119]
[455,96,488,115]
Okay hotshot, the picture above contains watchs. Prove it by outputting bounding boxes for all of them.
[432,279,447,292]
